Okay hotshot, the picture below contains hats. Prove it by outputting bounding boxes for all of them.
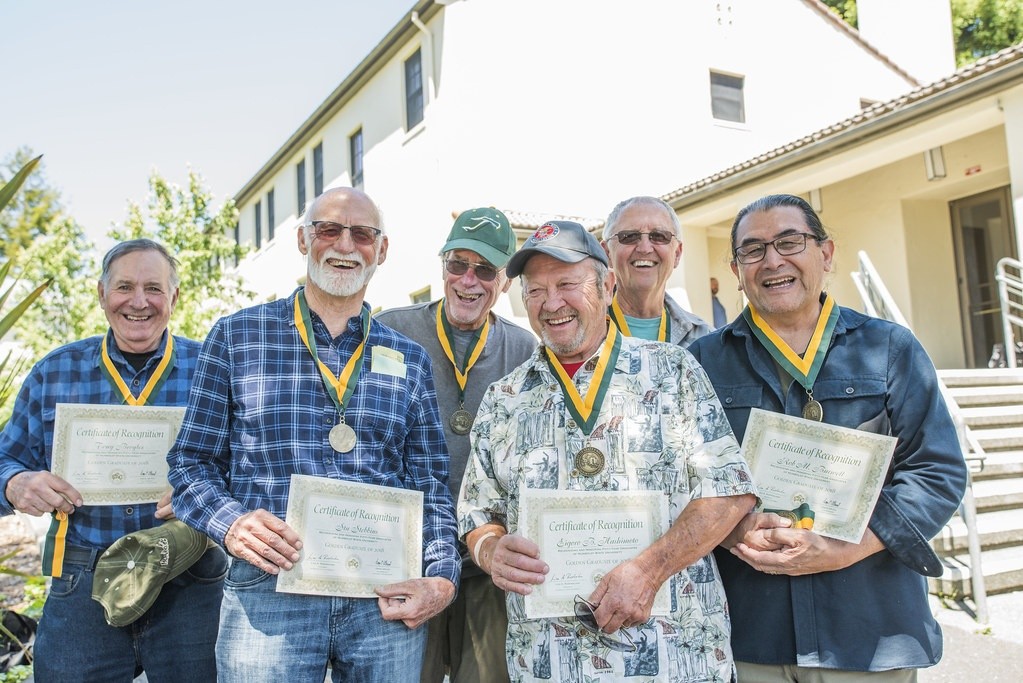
[438,207,516,268]
[505,221,609,278]
[91,517,208,628]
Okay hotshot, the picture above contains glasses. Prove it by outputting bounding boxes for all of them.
[304,220,381,245]
[573,594,637,652]
[442,259,506,282]
[606,229,680,244]
[735,233,823,264]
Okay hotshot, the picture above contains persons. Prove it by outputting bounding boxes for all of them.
[603,195,715,347]
[369,208,538,683]
[165,188,462,683]
[457,219,761,683]
[685,193,967,683]
[1,239,228,683]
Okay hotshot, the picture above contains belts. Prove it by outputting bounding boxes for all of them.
[63,537,219,566]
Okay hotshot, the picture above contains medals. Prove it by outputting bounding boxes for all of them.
[450,406,473,434]
[329,420,357,453]
[574,442,605,476]
[802,396,823,422]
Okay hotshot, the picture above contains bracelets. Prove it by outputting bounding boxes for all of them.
[474,532,497,567]
